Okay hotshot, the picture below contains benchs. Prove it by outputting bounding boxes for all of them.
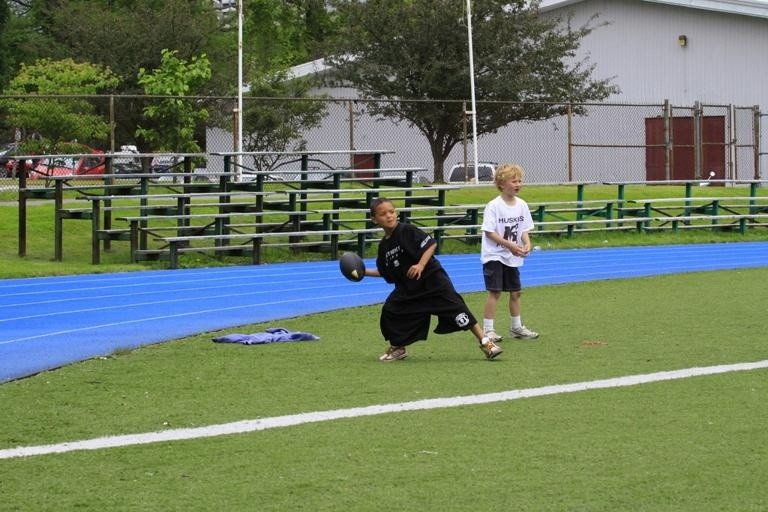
[465,178,767,250]
[2,149,470,268]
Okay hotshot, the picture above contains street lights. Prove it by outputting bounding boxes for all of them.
[220,0,245,184]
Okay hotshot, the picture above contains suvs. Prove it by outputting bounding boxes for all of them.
[446,161,503,183]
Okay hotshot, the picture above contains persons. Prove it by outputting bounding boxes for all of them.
[481,166,539,342]
[365,198,503,362]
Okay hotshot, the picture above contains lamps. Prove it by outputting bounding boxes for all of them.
[678,34,688,49]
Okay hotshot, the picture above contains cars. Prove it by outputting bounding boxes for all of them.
[0,142,184,181]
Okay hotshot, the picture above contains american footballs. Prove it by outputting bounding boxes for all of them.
[340,253,366,282]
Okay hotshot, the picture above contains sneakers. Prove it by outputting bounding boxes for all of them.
[484,329,504,342]
[378,346,408,362]
[510,324,539,339]
[478,341,503,360]
